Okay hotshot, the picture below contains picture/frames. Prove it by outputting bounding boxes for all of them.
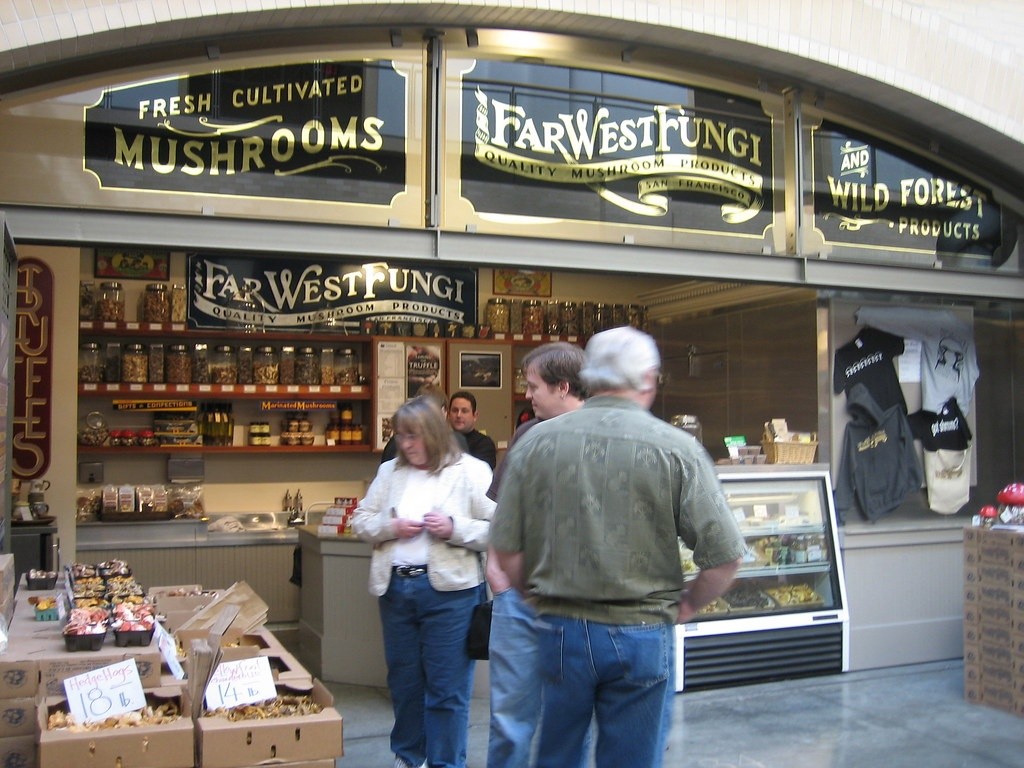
[93,248,171,282]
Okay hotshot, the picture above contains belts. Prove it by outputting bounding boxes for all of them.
[393,565,426,579]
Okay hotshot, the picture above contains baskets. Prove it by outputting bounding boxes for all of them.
[760,431,818,465]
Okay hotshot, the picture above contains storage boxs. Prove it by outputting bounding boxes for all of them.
[759,431,819,464]
[78,281,360,387]
[483,298,653,338]
[0,553,344,768]
[963,521,1024,719]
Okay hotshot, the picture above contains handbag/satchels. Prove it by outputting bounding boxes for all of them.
[467,601,494,660]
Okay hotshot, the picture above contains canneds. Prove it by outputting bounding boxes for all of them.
[249,402,363,445]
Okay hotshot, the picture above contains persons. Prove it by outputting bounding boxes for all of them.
[487,323,743,768]
[378,384,469,465]
[485,335,597,768]
[348,396,501,768]
[450,391,495,469]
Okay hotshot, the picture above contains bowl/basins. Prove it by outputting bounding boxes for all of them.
[63,562,155,652]
[26,569,58,590]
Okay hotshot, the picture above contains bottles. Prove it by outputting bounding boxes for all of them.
[77,410,109,447]
[79,279,358,385]
[514,368,529,394]
[486,298,647,336]
[200,401,233,446]
[789,534,828,562]
[282,489,292,512]
[294,489,302,511]
[670,415,702,446]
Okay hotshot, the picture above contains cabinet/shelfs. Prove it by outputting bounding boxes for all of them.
[672,462,851,693]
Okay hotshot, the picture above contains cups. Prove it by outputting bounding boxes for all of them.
[11,478,22,493]
[29,502,48,519]
[28,493,44,502]
[30,479,50,492]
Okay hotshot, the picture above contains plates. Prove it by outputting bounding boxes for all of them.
[11,516,56,527]
